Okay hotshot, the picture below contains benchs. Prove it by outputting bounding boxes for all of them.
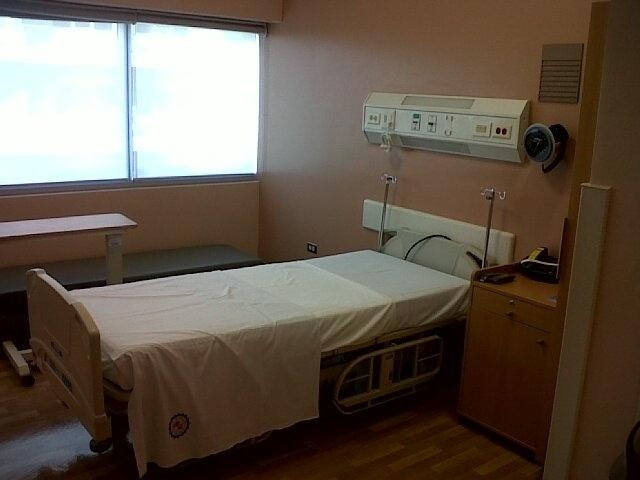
[0,243,267,301]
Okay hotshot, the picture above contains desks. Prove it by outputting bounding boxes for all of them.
[2,212,139,386]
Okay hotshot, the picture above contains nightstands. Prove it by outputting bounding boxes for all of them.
[457,257,559,465]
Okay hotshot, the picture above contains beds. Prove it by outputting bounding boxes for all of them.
[27,230,496,454]
